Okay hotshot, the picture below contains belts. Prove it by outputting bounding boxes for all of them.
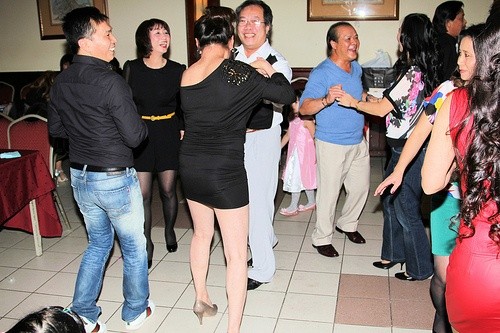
[70,162,134,172]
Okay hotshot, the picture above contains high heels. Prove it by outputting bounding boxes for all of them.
[148,228,177,268]
[373,261,417,281]
[193,300,218,325]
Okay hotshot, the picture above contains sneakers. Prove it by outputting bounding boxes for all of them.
[79,315,108,333]
[125,298,155,329]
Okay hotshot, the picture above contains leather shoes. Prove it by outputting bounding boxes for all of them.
[246,239,278,290]
[311,226,366,257]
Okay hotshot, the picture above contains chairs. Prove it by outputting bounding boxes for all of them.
[6,114,73,232]
[0,82,15,116]
[0,112,12,150]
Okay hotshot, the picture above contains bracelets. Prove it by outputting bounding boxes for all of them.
[355,101,363,111]
[321,97,327,108]
[366,94,373,103]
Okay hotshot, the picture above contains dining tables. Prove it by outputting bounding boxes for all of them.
[0,149,56,257]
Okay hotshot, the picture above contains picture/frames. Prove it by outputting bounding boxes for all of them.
[306,0,399,21]
[37,0,109,41]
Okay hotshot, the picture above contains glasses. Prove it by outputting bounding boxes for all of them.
[236,16,269,28]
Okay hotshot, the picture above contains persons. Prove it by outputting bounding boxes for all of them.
[278,88,319,218]
[298,22,380,256]
[333,13,438,283]
[373,0,500,333]
[24,56,74,187]
[121,17,187,270]
[230,0,292,289]
[5,306,86,333]
[180,5,296,333]
[46,5,156,333]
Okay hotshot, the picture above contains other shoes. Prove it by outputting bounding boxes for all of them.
[279,203,316,216]
